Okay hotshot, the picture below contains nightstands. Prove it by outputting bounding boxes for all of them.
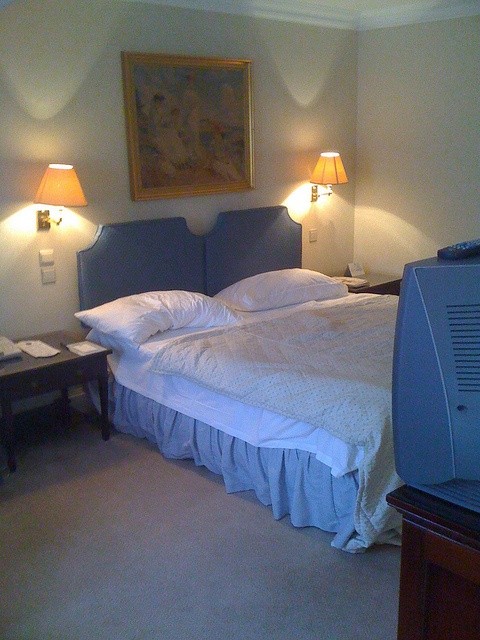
[0,330,113,472]
[330,273,402,295]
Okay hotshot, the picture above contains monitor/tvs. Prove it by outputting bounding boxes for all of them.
[392,257,479,516]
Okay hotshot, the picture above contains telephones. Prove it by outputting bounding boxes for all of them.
[0,336,22,362]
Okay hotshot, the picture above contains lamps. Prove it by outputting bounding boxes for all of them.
[311,153,349,202]
[33,165,88,232]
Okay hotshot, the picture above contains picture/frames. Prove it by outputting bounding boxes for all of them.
[121,51,255,201]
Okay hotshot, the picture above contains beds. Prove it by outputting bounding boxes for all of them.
[75,206,403,554]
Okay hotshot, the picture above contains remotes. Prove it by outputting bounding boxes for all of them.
[437,238,480,260]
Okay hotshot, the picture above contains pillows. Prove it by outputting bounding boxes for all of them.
[74,290,244,351]
[211,267,350,313]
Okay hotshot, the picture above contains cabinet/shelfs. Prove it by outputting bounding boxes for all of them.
[385,486,479,636]
